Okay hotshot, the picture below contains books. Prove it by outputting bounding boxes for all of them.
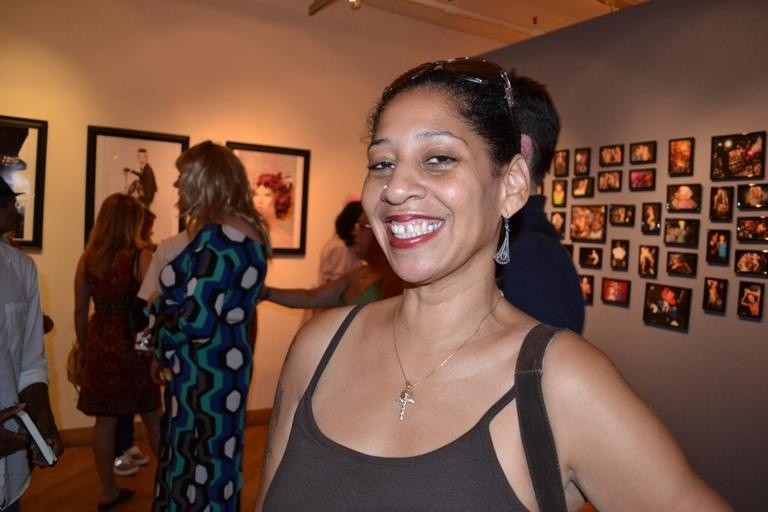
[11,409,57,466]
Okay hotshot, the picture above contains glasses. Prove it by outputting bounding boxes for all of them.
[383,58,521,153]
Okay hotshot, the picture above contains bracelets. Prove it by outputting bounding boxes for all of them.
[263,286,271,298]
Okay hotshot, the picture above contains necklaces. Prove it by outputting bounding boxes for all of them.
[392,291,506,422]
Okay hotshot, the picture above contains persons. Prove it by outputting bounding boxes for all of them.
[298,199,363,329]
[257,212,404,310]
[2,230,55,333]
[537,135,768,328]
[253,57,734,512]
[252,170,294,249]
[123,149,159,209]
[133,139,270,512]
[68,192,165,510]
[0,177,64,510]
[111,208,158,477]
[495,66,585,334]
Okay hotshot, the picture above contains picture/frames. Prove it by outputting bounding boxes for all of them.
[665,183,702,214]
[0,112,50,252]
[663,217,700,250]
[736,183,767,212]
[734,248,766,279]
[708,185,735,224]
[573,147,592,176]
[596,169,623,193]
[709,130,766,182]
[571,176,595,199]
[550,210,567,241]
[610,238,630,273]
[598,143,624,167]
[224,139,312,258]
[561,243,575,263]
[666,250,698,279]
[701,275,728,317]
[736,280,765,323]
[705,228,732,268]
[629,140,657,165]
[553,148,570,178]
[667,136,696,177]
[551,179,568,208]
[642,281,692,335]
[82,123,192,267]
[736,214,767,245]
[639,201,662,236]
[578,274,595,306]
[637,244,660,280]
[578,246,603,270]
[628,168,657,192]
[600,276,632,309]
[609,202,636,228]
[569,203,608,245]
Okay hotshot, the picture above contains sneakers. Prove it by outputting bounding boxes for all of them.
[113,446,148,476]
